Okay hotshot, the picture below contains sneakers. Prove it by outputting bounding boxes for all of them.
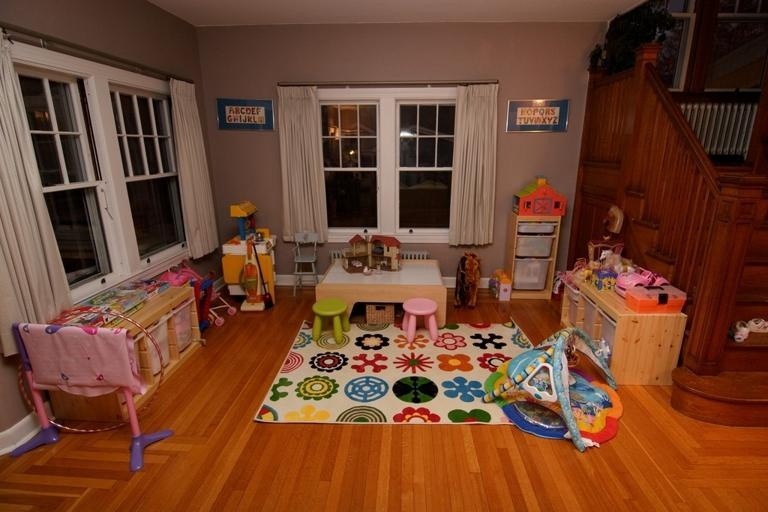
[735,320,748,339]
[747,319,767,333]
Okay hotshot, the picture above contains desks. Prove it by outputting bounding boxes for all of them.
[221,234,279,304]
[316,259,447,329]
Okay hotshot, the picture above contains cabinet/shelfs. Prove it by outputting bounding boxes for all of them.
[44,283,206,423]
[292,231,319,297]
[506,211,562,300]
[560,269,688,386]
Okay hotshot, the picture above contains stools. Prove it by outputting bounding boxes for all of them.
[312,298,439,344]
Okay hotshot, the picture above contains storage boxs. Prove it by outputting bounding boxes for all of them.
[513,221,557,291]
[113,297,195,405]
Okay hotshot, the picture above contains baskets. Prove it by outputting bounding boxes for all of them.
[365,304,394,324]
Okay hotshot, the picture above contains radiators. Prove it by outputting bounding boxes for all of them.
[672,91,759,160]
[329,248,431,263]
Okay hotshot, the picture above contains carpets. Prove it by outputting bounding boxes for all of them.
[253,316,534,424]
